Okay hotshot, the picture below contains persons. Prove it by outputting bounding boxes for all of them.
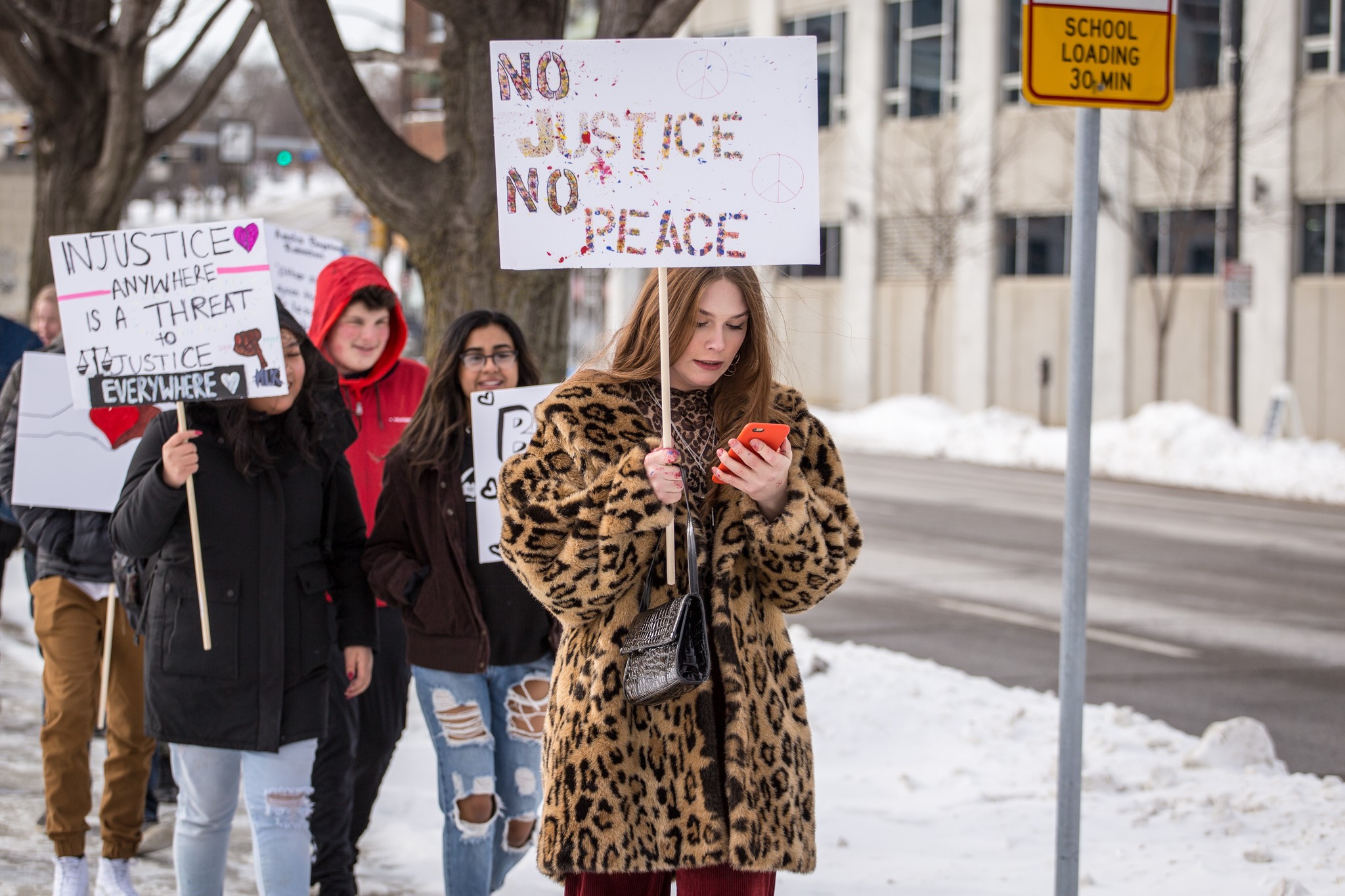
[304,256,432,896]
[498,262,862,896]
[0,283,178,896]
[107,293,374,896]
[367,310,563,896]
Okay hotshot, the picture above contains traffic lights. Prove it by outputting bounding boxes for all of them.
[276,149,292,167]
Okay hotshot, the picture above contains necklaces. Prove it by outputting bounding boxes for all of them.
[642,378,717,526]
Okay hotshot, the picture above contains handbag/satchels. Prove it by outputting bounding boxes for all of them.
[619,466,711,706]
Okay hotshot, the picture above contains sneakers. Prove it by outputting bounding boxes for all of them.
[95,857,137,896]
[51,854,87,896]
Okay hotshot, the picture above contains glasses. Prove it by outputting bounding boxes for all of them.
[461,349,522,371]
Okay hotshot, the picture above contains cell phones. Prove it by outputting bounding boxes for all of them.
[711,422,790,485]
